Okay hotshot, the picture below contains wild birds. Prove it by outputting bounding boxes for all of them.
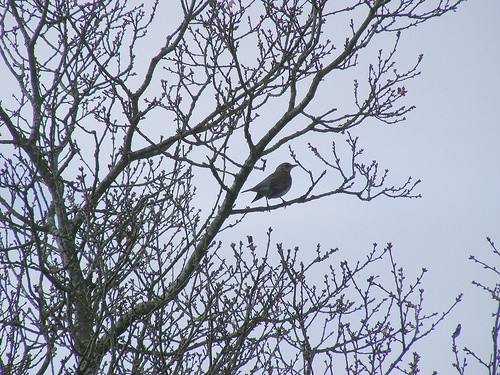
[240,162,298,212]
